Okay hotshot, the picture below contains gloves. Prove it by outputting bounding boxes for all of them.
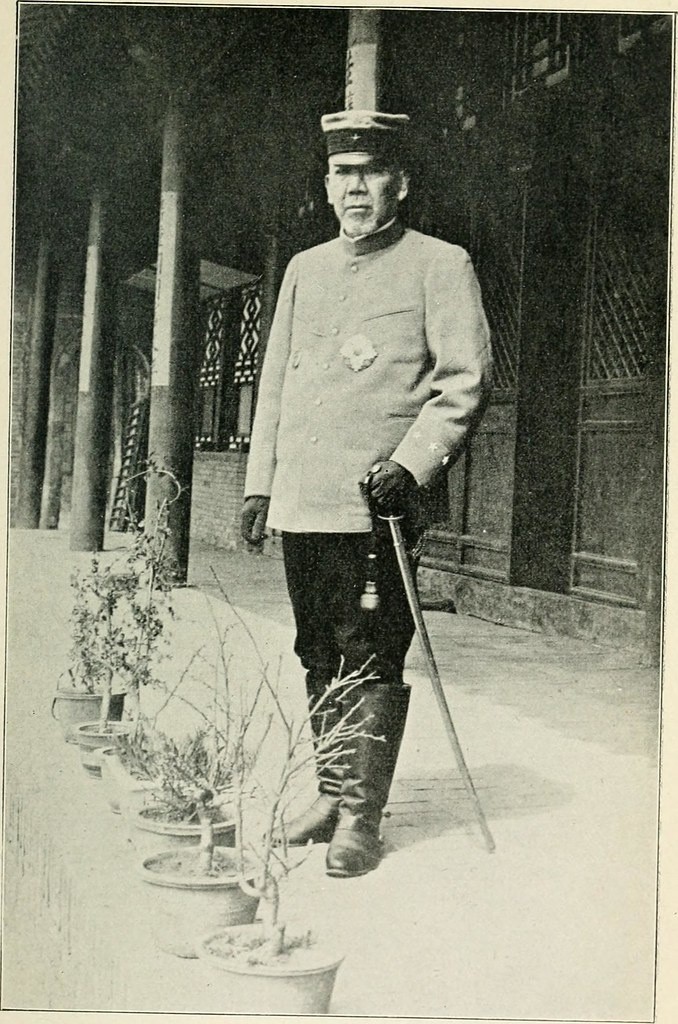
[240,496,270,547]
[359,460,417,517]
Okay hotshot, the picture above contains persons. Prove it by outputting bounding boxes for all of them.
[240,112,495,878]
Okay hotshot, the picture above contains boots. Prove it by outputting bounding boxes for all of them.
[261,701,344,847]
[325,682,412,877]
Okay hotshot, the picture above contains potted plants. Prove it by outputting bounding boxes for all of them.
[47,444,392,1014]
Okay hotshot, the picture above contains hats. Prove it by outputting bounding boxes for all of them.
[320,109,411,165]
